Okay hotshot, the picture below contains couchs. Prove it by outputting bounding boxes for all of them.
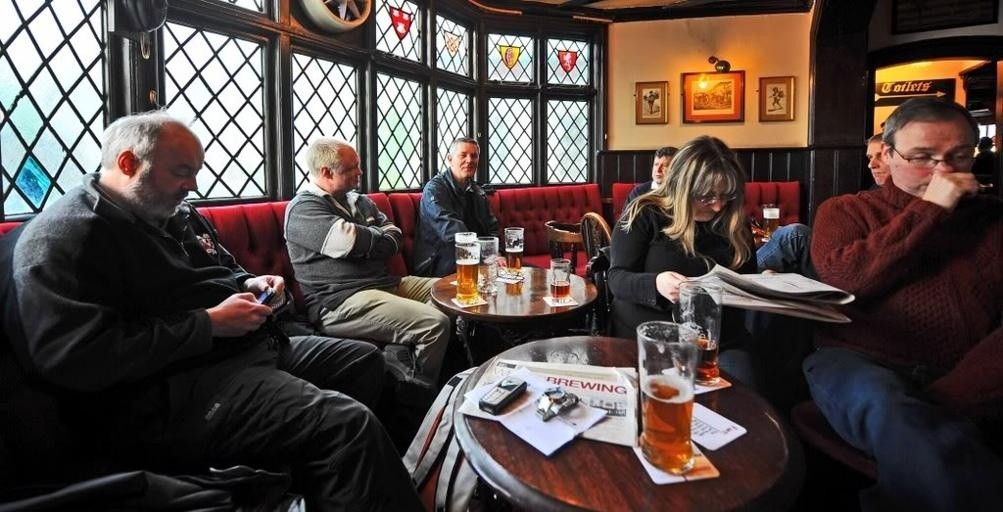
[613,179,805,228]
[389,176,609,279]
[0,188,445,512]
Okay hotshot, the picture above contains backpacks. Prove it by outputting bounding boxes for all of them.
[402,366,479,512]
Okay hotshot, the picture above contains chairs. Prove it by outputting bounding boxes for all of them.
[580,244,638,337]
[788,396,885,511]
[582,208,615,253]
[542,218,589,278]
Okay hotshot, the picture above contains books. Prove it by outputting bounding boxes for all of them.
[456,366,609,459]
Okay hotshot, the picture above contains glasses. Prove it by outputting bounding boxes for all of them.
[701,196,736,204]
[891,145,976,169]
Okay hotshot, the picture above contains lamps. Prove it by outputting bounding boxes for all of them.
[708,56,730,73]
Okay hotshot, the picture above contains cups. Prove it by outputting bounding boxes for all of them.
[636,320,699,473]
[550,258,571,304]
[452,226,525,304]
[761,202,782,238]
[506,281,523,296]
[678,281,724,386]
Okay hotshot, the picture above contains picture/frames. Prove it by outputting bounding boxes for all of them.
[756,74,797,122]
[633,80,670,126]
[680,71,746,124]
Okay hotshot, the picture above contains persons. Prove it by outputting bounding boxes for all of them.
[621,146,680,218]
[10,108,427,508]
[407,136,501,277]
[605,137,779,387]
[753,132,893,280]
[972,136,1000,182]
[280,136,466,387]
[805,91,1003,510]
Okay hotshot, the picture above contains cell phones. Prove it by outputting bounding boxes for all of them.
[256,287,276,305]
[478,375,526,416]
